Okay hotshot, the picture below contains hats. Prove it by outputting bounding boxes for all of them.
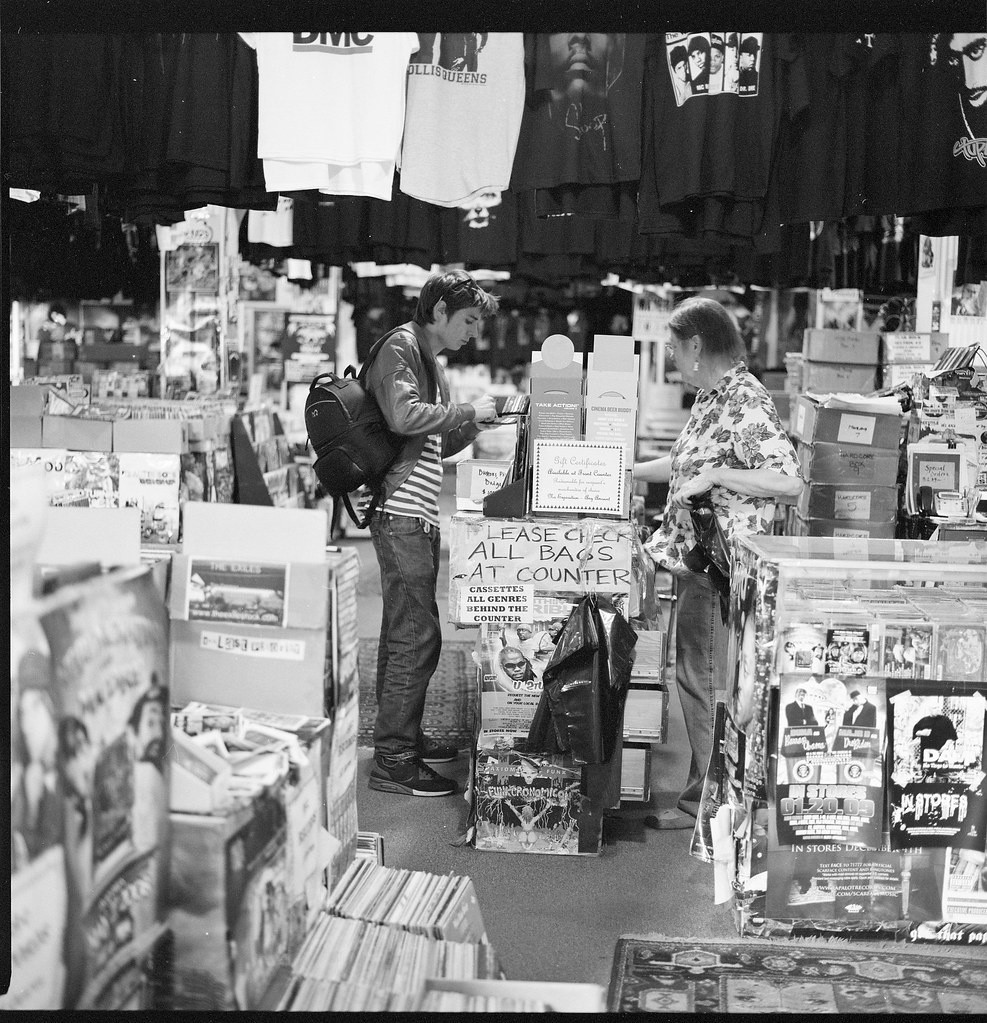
[850,691,860,699]
[517,624,532,632]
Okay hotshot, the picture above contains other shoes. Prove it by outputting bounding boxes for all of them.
[645,807,697,829]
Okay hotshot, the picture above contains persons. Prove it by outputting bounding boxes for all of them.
[499,620,565,682]
[549,33,625,100]
[631,296,804,829]
[844,691,877,727]
[12,649,167,876]
[911,701,958,783]
[365,268,509,796]
[670,34,761,102]
[786,688,817,726]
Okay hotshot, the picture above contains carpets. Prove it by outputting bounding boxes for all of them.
[607,930,987,1014]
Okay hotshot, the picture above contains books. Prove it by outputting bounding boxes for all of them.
[768,584,987,853]
[270,827,550,1013]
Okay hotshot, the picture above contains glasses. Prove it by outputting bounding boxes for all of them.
[504,661,526,668]
[437,278,477,304]
[801,703,803,711]
[665,332,701,355]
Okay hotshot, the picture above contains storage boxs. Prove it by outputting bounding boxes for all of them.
[136,544,360,1011]
[10,385,188,455]
[456,458,514,510]
[801,328,949,394]
[785,395,904,539]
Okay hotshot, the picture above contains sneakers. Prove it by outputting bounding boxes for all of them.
[367,756,458,796]
[417,735,459,763]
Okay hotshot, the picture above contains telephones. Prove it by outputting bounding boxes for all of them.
[917,486,969,517]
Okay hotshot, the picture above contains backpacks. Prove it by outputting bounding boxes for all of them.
[305,328,437,541]
